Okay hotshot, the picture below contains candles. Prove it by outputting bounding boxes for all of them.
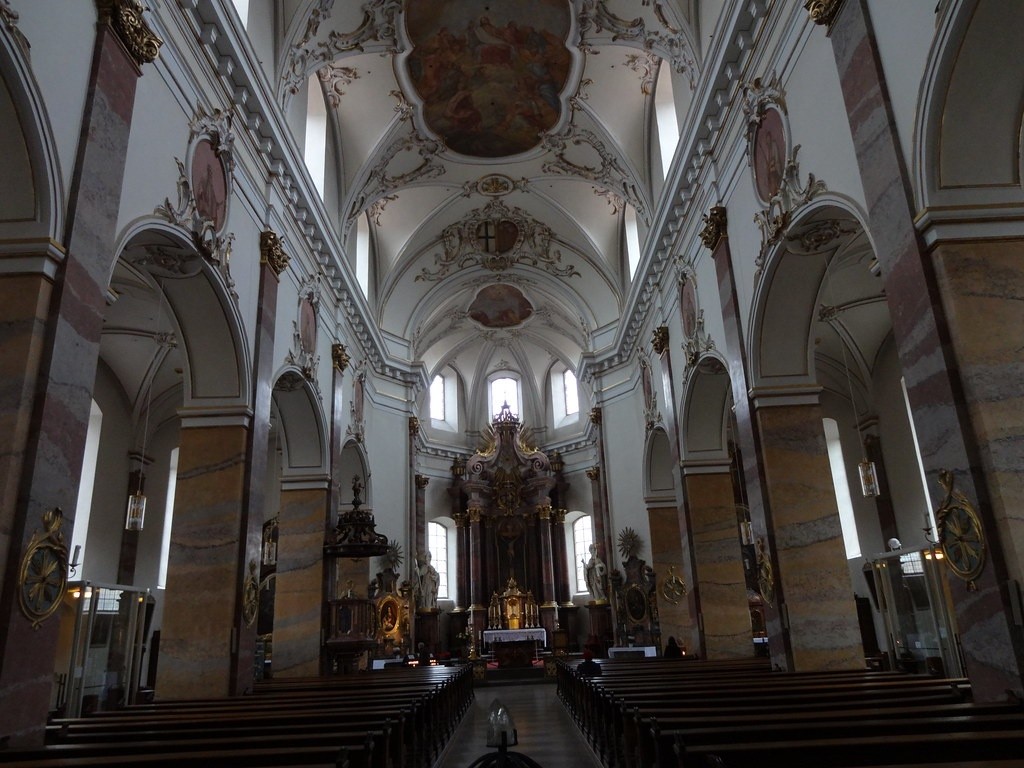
[470,604,473,624]
[478,631,481,640]
[543,630,547,647]
[530,604,533,614]
[488,607,491,618]
[923,508,933,528]
[498,604,501,615]
[72,544,81,565]
[536,605,539,615]
[555,601,558,615]
[493,606,497,619]
[525,604,528,615]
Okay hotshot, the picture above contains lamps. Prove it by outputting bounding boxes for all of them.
[819,256,880,498]
[124,277,167,532]
[727,409,754,548]
[264,391,281,566]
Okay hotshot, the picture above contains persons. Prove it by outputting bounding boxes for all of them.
[418,643,432,666]
[383,606,396,627]
[577,649,602,718]
[664,637,683,661]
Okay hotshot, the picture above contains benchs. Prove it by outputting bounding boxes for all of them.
[555,651,1024,768]
[0,662,475,768]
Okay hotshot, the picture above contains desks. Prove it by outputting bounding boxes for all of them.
[484,628,545,669]
